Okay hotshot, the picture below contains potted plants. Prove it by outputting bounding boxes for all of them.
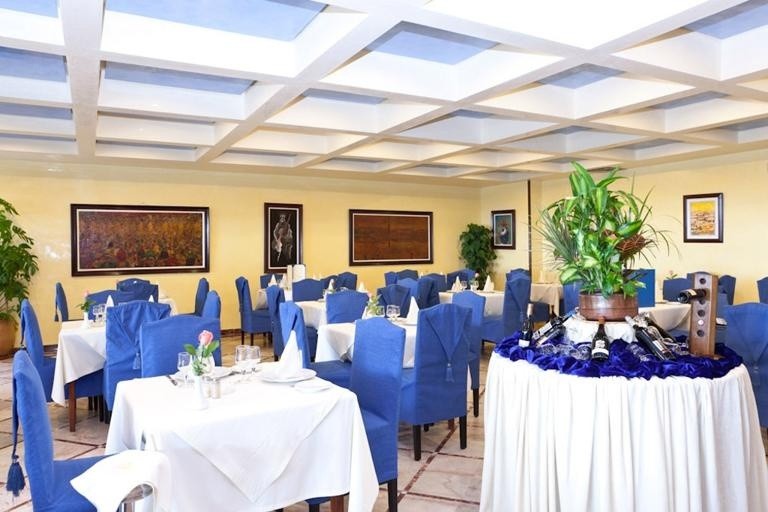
[533,161,671,322]
[0,196,40,361]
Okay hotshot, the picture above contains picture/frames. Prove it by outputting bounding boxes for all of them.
[69,202,211,278]
[491,208,518,251]
[682,191,725,245]
[348,209,435,267]
[264,200,306,275]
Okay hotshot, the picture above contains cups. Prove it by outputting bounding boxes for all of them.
[246,345,262,382]
[98,303,107,326]
[235,345,249,383]
[461,281,467,291]
[468,279,474,291]
[177,352,192,386]
[386,303,395,321]
[375,305,385,318]
[394,306,401,323]
[92,305,101,325]
[474,280,479,293]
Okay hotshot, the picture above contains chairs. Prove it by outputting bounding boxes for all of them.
[506,268,531,279]
[137,312,222,378]
[718,274,737,306]
[260,274,284,288]
[481,274,530,357]
[200,290,222,317]
[719,302,768,427]
[117,278,161,305]
[54,281,69,322]
[86,289,118,321]
[292,279,324,302]
[104,302,174,427]
[662,278,694,304]
[376,284,411,318]
[414,276,440,310]
[561,277,588,315]
[452,290,486,417]
[277,301,354,389]
[756,274,768,303]
[447,268,476,290]
[320,275,337,290]
[335,272,358,291]
[235,276,272,346]
[384,271,398,286]
[399,302,474,461]
[274,317,406,512]
[18,298,105,421]
[5,351,155,512]
[421,274,445,292]
[194,278,210,312]
[396,269,419,297]
[265,285,319,362]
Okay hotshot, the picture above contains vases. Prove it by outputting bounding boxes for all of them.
[189,355,215,375]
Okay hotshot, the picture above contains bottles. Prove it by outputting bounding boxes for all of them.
[528,312,574,344]
[591,316,609,360]
[678,288,706,303]
[519,303,533,348]
[535,318,575,351]
[625,316,675,362]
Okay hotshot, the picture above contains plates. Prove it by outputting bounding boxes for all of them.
[255,367,319,384]
[205,366,233,379]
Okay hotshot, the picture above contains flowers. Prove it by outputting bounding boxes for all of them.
[186,329,220,359]
